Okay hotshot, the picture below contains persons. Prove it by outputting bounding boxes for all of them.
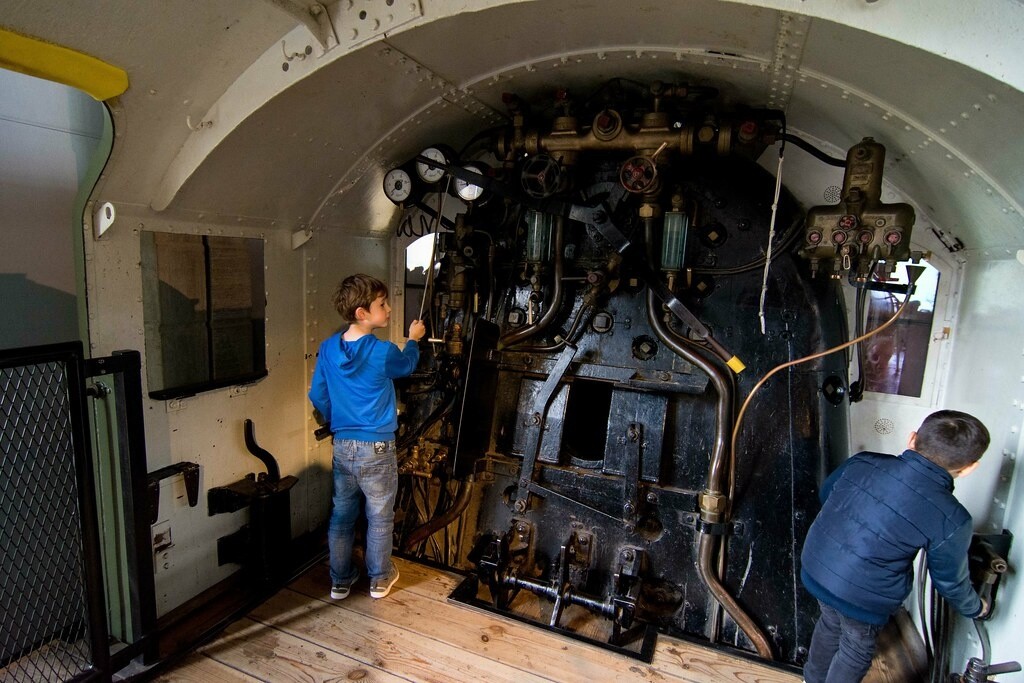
[306,271,427,600]
[797,409,991,683]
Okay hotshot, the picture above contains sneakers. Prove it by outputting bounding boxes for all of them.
[330,567,361,599]
[370,562,399,598]
